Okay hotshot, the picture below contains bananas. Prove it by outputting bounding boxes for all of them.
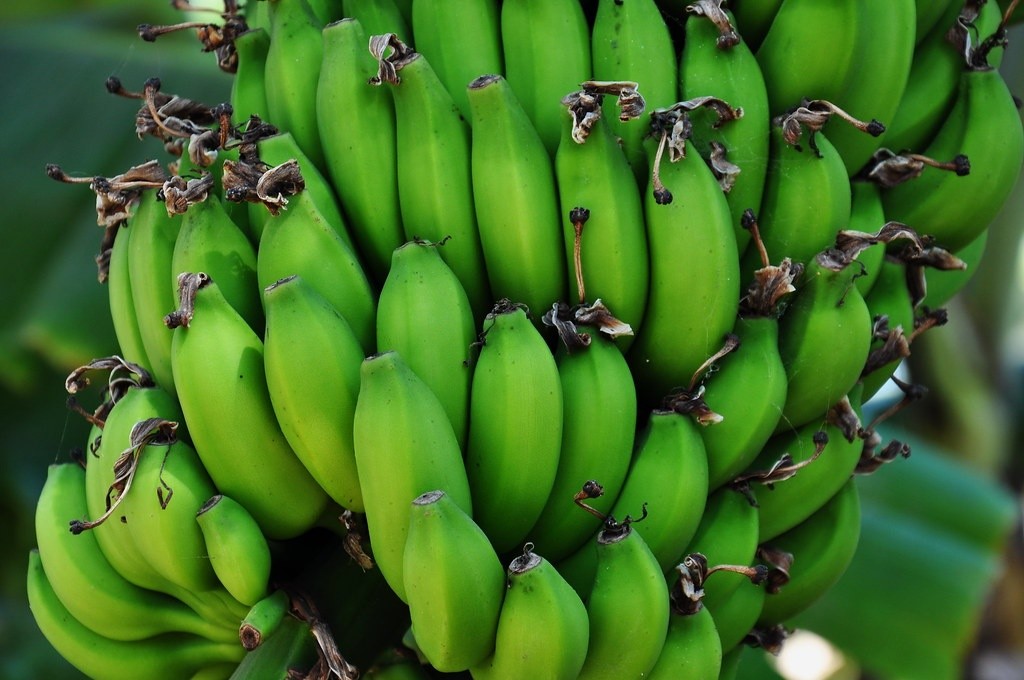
[23,1,1024,680]
